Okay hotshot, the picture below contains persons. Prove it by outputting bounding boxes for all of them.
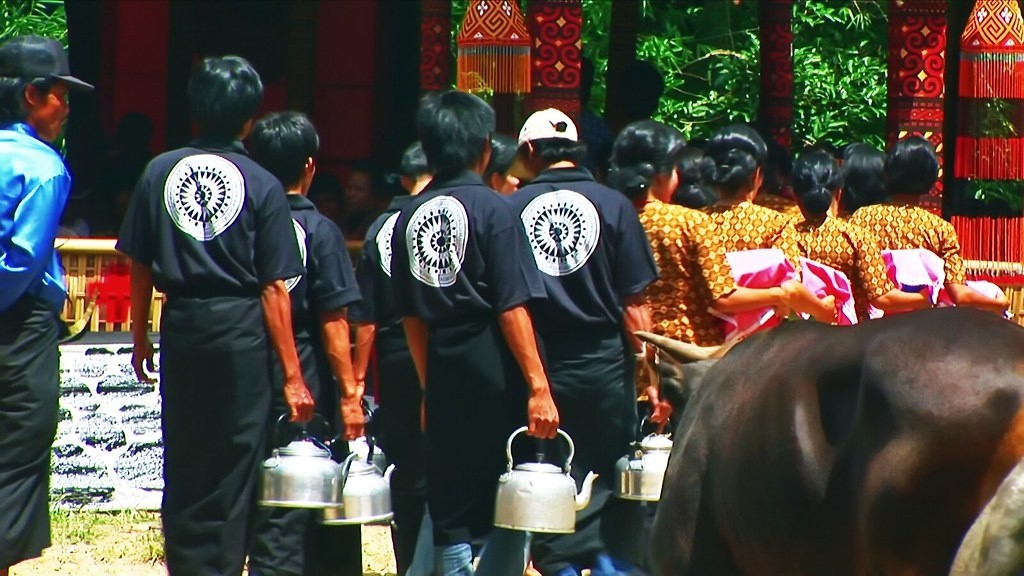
[115,54,314,576]
[0,37,94,576]
[67,71,157,239]
[393,89,560,576]
[580,57,663,178]
[245,107,970,576]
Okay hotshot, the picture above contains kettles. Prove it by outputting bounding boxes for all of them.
[254,412,358,508]
[492,426,600,534]
[612,413,672,500]
[315,430,396,525]
[349,435,386,476]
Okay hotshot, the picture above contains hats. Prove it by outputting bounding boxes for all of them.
[516,108,580,142]
[2,34,94,93]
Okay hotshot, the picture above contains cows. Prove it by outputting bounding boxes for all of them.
[633,311,1024,576]
